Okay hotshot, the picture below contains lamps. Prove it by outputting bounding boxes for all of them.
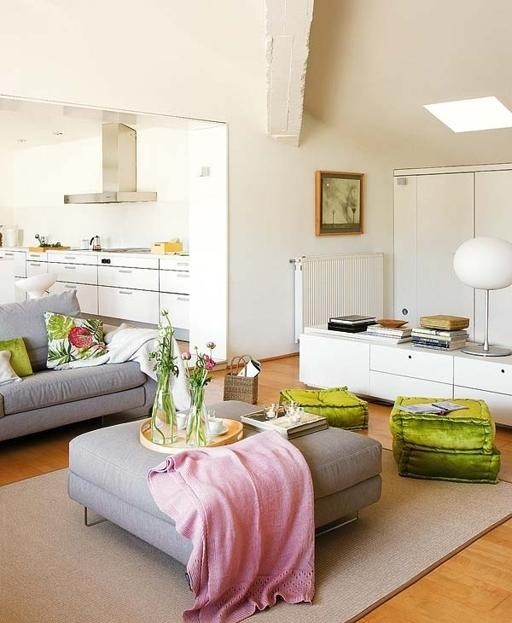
[452,237,510,357]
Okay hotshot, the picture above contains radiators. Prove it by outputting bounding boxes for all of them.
[290,252,386,344]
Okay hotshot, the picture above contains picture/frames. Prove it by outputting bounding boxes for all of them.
[315,168,366,238]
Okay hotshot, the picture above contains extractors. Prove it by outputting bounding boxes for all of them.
[61,120,159,205]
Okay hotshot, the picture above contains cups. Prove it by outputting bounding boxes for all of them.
[174,414,188,430]
[81,239,90,249]
[207,417,224,432]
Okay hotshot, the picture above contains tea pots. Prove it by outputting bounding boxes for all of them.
[89,235,100,251]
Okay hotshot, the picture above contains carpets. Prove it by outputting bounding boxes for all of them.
[1,445,512,621]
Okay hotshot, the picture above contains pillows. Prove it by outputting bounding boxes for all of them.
[0,350,21,384]
[43,310,110,369]
[0,337,33,378]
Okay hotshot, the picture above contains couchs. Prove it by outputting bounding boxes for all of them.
[279,387,369,441]
[0,290,179,442]
[390,395,500,483]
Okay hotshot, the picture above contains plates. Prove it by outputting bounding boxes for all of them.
[375,319,409,329]
[207,425,230,437]
[139,417,245,455]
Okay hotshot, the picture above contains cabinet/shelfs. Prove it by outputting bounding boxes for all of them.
[26,254,50,299]
[97,252,159,327]
[159,256,190,342]
[0,249,27,305]
[388,164,511,349]
[297,323,511,429]
[47,250,98,319]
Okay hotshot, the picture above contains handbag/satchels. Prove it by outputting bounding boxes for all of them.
[222,355,258,405]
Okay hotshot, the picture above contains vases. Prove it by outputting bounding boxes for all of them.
[185,387,210,445]
[152,373,179,444]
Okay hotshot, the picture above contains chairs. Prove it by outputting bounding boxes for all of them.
[16,270,59,299]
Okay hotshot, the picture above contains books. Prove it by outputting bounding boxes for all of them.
[328,314,375,333]
[239,406,328,441]
[409,314,470,351]
[400,400,467,415]
[367,325,415,343]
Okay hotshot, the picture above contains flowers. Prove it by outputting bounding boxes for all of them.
[148,307,180,445]
[181,342,216,445]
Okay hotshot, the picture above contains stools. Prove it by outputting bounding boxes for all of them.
[67,397,381,571]
